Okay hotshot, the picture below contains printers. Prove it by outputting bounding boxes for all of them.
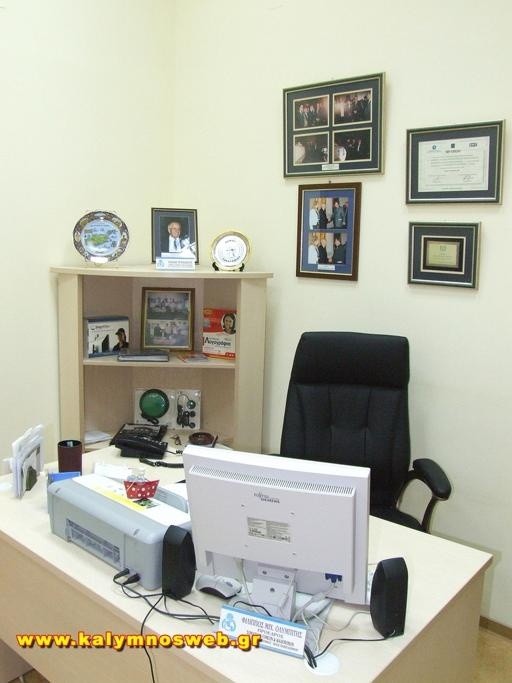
[48,471,188,591]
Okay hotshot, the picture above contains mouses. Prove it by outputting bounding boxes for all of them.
[196,574,241,599]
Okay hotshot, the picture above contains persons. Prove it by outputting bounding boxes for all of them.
[310,199,320,228]
[295,136,328,164]
[334,130,370,161]
[335,90,372,123]
[318,239,328,263]
[308,235,320,263]
[332,238,346,264]
[221,312,236,334]
[162,221,186,252]
[295,99,329,128]
[113,328,128,350]
[144,319,190,347]
[146,290,191,319]
[331,201,345,228]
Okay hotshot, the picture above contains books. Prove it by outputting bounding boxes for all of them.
[119,352,169,361]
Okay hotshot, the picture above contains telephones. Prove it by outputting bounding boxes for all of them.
[115,435,169,460]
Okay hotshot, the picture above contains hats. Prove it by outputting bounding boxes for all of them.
[116,328,125,334]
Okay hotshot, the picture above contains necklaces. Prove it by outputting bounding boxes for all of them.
[317,204,330,227]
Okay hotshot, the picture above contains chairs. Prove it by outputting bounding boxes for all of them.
[268,330,452,534]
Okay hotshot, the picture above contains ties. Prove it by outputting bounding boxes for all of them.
[174,240,177,248]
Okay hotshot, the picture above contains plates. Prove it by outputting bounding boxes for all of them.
[72,210,129,263]
[208,228,252,269]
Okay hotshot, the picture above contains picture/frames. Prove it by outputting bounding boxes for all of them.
[150,207,199,264]
[295,181,364,281]
[407,221,481,289]
[3,424,47,499]
[282,70,385,177]
[140,285,195,355]
[407,117,506,205]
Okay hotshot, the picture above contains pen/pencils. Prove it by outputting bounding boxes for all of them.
[68,441,72,446]
[67,440,70,448]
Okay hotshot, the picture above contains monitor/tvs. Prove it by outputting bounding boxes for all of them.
[182,445,371,622]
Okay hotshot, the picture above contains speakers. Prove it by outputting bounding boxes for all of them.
[372,557,408,638]
[162,525,194,598]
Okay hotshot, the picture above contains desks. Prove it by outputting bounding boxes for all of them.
[0,430,497,683]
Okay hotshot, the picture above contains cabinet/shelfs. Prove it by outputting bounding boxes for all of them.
[47,259,274,456]
